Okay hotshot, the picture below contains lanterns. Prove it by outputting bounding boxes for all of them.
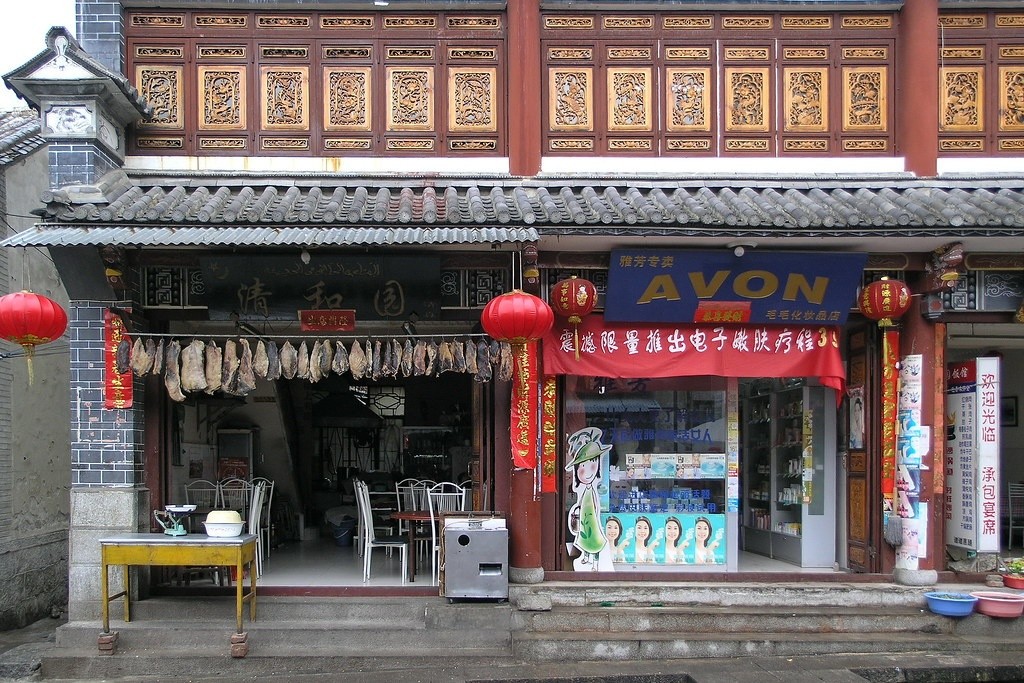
[858,275,912,366]
[480,290,554,402]
[0,290,68,388]
[550,275,598,361]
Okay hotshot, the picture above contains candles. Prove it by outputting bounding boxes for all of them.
[970,591,1024,618]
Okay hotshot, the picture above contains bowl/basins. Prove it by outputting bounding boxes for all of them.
[924,592,978,616]
[969,592,1024,617]
[205,510,242,523]
[1002,574,1024,588]
[202,521,247,537]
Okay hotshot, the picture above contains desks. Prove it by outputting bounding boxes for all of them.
[99,533,259,634]
[391,511,439,583]
[177,506,242,586]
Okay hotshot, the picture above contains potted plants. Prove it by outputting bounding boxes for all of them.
[924,592,978,616]
[1004,555,1024,589]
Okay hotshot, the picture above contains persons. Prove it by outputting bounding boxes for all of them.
[851,398,862,442]
[626,468,634,478]
[634,516,659,563]
[676,468,684,477]
[664,516,690,565]
[694,516,720,564]
[642,454,651,465]
[692,454,700,467]
[606,516,630,563]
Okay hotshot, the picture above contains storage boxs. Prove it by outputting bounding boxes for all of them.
[620,486,709,513]
[739,399,824,535]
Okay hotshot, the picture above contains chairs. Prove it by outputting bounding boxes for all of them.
[184,476,275,585]
[351,477,488,584]
[1007,482,1024,552]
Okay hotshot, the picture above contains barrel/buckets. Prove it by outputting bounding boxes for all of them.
[331,516,356,546]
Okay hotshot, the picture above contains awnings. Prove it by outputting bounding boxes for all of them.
[565,396,662,414]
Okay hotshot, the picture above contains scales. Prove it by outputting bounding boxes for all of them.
[153,505,197,537]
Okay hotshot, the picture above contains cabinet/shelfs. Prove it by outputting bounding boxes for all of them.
[744,392,777,559]
[773,385,838,569]
[739,398,747,550]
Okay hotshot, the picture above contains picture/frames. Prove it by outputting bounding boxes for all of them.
[1001,396,1018,427]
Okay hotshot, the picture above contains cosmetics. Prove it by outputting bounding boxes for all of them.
[749,507,771,530]
[782,455,803,473]
[751,433,770,447]
[750,402,771,423]
[753,462,770,473]
[619,485,651,512]
[774,521,802,535]
[780,399,803,417]
[777,418,804,446]
[663,487,705,511]
[777,483,803,505]
[749,480,769,501]
